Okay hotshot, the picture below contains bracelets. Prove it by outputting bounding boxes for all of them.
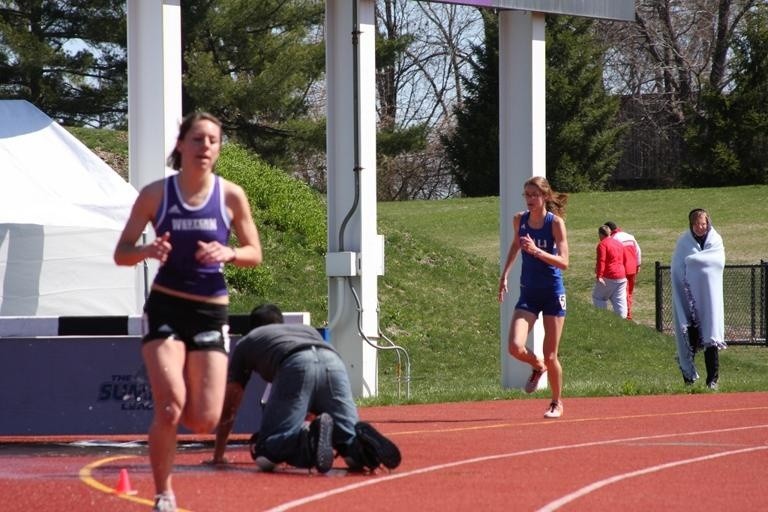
[228,247,237,264]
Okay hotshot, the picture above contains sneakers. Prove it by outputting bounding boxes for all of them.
[309,412,333,471]
[708,381,717,392]
[524,365,545,394]
[354,420,401,469]
[544,401,563,419]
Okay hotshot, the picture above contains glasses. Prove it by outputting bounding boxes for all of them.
[522,193,541,200]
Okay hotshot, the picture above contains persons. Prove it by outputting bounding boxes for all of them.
[200,302,402,474]
[604,221,642,320]
[114,108,264,512]
[497,176,570,418]
[669,207,726,391]
[591,225,628,320]
